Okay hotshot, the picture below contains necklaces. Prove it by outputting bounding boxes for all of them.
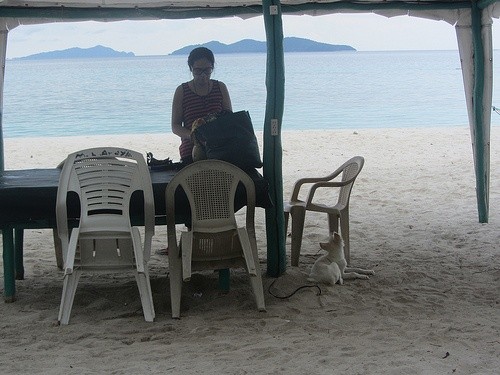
[192,79,210,94]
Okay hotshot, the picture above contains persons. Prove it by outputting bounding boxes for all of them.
[171,47,232,231]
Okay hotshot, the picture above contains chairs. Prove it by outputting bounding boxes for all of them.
[56,146,155,324]
[284,156,365,266]
[164,159,266,319]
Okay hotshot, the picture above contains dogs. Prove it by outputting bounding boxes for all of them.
[307,232,375,285]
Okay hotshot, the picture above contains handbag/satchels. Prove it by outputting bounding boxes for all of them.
[193,109,263,171]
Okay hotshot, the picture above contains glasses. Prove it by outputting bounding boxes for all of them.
[192,67,213,75]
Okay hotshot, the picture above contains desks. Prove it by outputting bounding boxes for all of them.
[0,165,245,304]
